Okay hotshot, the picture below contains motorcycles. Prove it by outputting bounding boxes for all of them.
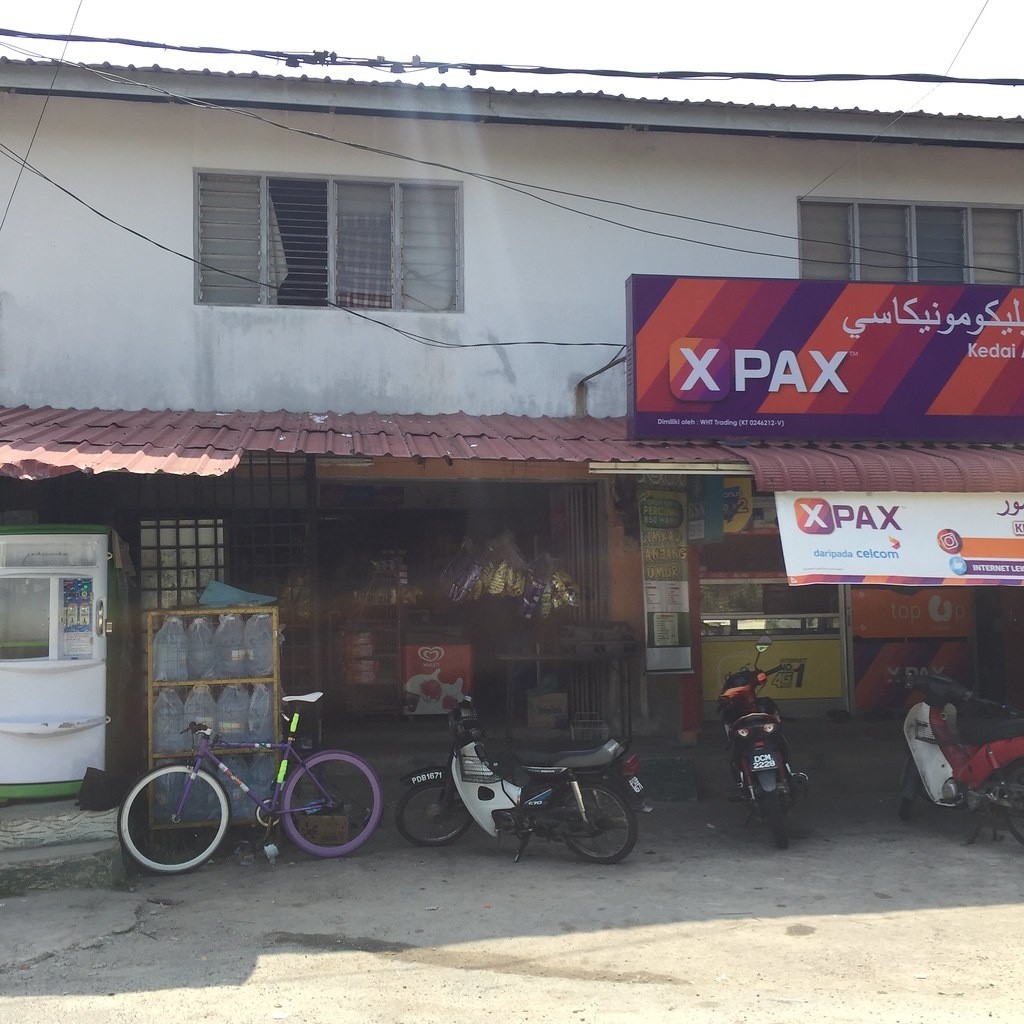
[898,669,1024,848]
[395,692,652,865]
[712,634,810,851]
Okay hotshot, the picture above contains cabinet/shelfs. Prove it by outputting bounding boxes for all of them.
[144,605,286,856]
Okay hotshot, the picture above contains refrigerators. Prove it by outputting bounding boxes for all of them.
[403,627,473,720]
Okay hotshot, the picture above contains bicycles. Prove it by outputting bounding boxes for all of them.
[118,692,384,875]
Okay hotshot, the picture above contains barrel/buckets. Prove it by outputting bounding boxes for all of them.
[153,613,275,823]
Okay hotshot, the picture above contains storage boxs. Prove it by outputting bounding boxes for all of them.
[526,688,569,729]
[570,718,609,741]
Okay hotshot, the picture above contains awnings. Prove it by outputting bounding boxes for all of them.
[0,405,1024,490]
